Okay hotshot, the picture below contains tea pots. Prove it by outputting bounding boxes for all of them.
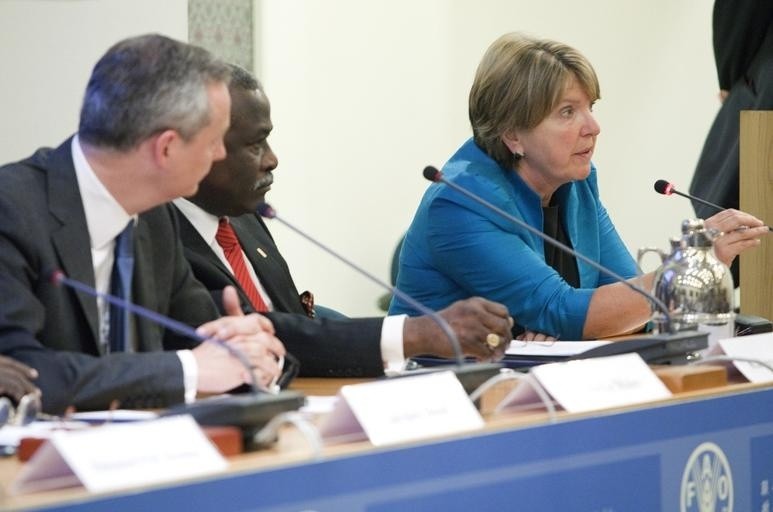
[636,216,735,365]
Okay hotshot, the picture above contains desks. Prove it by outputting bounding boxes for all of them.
[1,374,771,509]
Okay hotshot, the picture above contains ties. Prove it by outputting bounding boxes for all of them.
[214,218,269,316]
[105,217,136,356]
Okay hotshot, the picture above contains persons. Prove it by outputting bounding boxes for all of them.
[387,31,770,350]
[690,0,771,280]
[163,61,513,377]
[1,352,43,417]
[1,35,292,407]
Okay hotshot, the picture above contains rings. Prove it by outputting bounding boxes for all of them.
[485,332,497,351]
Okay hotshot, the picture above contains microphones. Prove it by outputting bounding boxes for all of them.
[654,178,772,333]
[257,202,505,410]
[423,165,710,365]
[40,267,306,451]
